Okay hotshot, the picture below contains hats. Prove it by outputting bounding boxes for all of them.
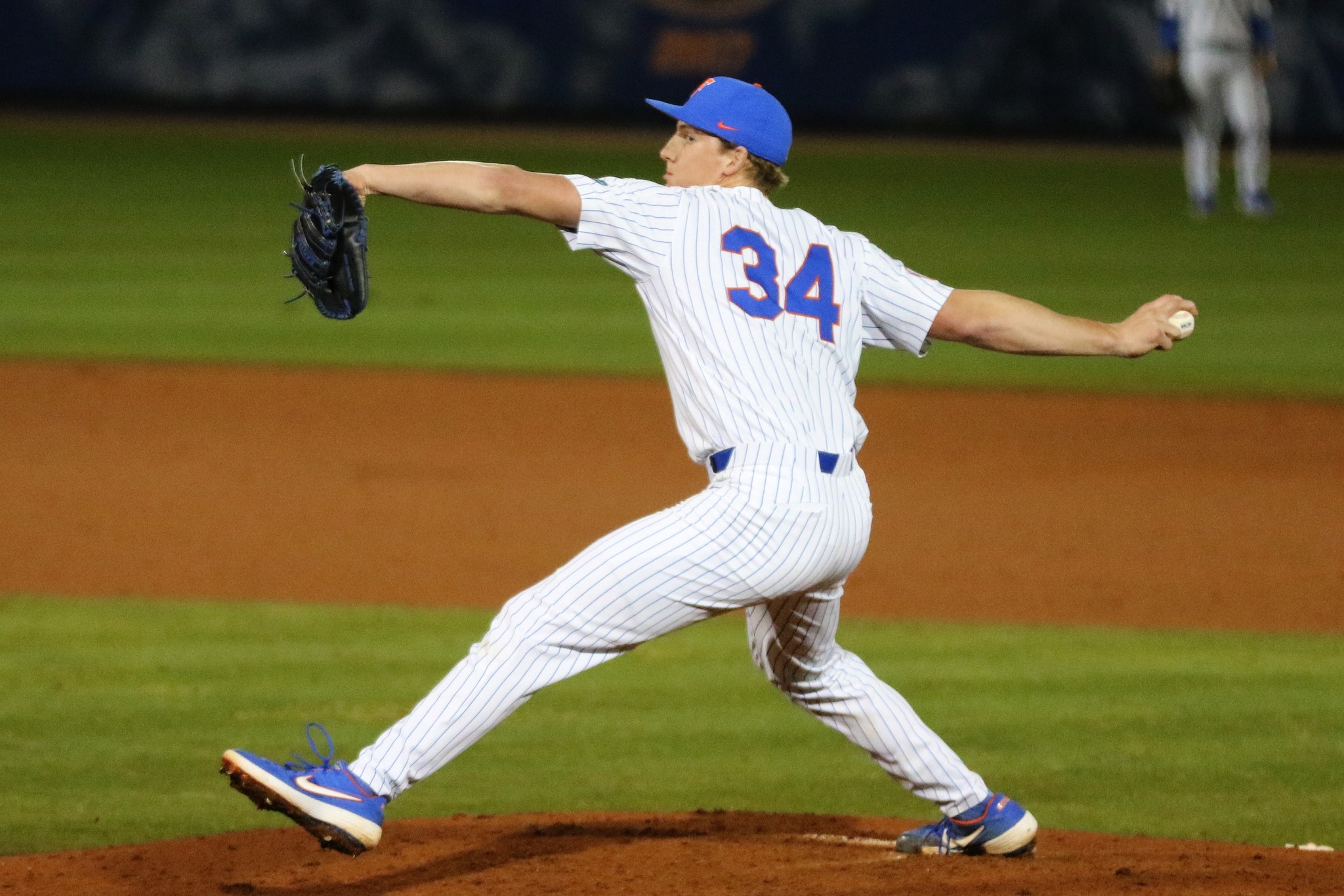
[644,76,792,166]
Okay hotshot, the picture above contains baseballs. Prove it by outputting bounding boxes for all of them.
[1168,310,1195,339]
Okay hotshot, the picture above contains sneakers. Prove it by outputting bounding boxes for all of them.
[895,793,1039,855]
[218,723,390,854]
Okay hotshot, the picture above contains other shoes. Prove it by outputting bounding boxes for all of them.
[1238,191,1270,218]
[1190,195,1219,226]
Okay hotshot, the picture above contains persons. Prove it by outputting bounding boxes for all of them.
[1156,0,1278,213]
[219,77,1201,854]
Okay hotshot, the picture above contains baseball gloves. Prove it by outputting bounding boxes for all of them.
[289,162,369,320]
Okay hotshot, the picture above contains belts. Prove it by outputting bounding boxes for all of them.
[710,445,837,473]
